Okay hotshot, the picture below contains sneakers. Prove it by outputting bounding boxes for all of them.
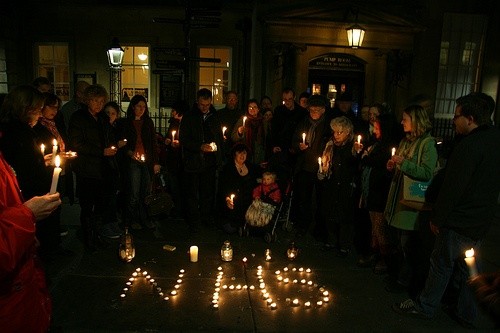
[392,298,433,320]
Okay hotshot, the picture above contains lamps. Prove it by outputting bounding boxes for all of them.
[346,9,366,50]
[106,37,126,72]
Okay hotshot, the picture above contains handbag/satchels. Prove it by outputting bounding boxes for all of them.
[148,173,168,209]
[60,171,81,225]
[245,200,276,227]
[399,137,445,210]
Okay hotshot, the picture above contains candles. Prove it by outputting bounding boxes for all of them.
[243,116,247,127]
[190,246,198,262]
[222,126,227,136]
[231,194,235,208]
[172,131,176,141]
[318,157,322,172]
[358,135,361,144]
[40,144,44,156]
[392,148,397,156]
[302,132,306,145]
[50,154,62,195]
[51,139,58,156]
[464,249,478,275]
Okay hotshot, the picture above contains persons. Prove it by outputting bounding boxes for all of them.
[0,75,500,332]
[384,104,438,294]
[179,89,216,232]
[0,147,61,333]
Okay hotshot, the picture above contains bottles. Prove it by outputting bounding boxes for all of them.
[221,239,233,262]
[286,244,298,262]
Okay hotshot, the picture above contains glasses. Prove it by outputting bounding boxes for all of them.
[334,130,343,135]
[47,105,59,111]
[283,98,293,101]
[249,107,257,110]
[308,107,323,113]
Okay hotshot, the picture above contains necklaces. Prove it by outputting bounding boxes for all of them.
[236,161,246,173]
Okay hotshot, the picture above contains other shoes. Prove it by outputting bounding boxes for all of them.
[101,222,123,238]
[382,275,403,294]
[375,259,387,274]
[333,243,353,256]
[318,238,340,254]
[356,256,368,268]
